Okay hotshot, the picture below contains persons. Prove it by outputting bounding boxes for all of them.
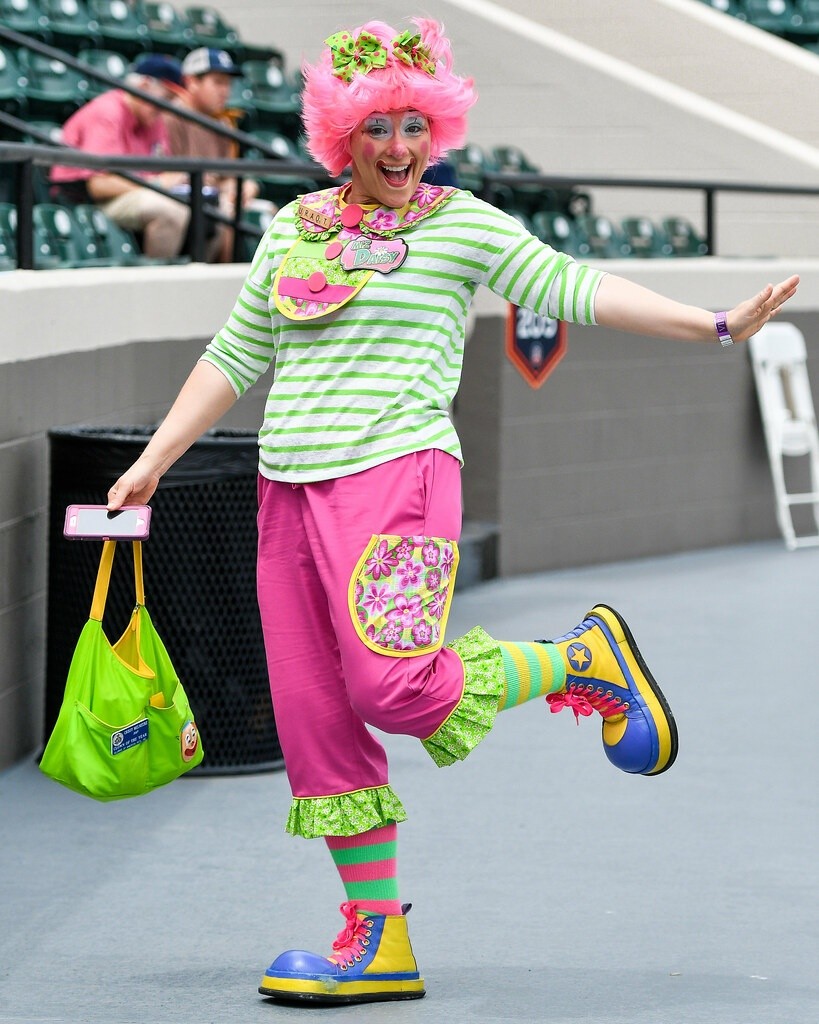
[103,22,801,1001]
[43,47,266,266]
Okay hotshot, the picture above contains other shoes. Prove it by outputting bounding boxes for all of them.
[534,604,679,777]
[258,902,426,1003]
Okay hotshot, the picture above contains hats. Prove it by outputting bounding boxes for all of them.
[126,52,192,103]
[181,48,245,77]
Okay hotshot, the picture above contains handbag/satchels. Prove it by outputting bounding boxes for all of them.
[39,539,204,803]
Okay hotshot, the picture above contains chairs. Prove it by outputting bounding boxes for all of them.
[1,0,818,271]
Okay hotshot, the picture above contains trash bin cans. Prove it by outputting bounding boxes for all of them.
[33,424,287,780]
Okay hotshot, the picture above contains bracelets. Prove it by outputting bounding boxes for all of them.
[714,310,734,350]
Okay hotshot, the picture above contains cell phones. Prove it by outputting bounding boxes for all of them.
[64,505,152,541]
